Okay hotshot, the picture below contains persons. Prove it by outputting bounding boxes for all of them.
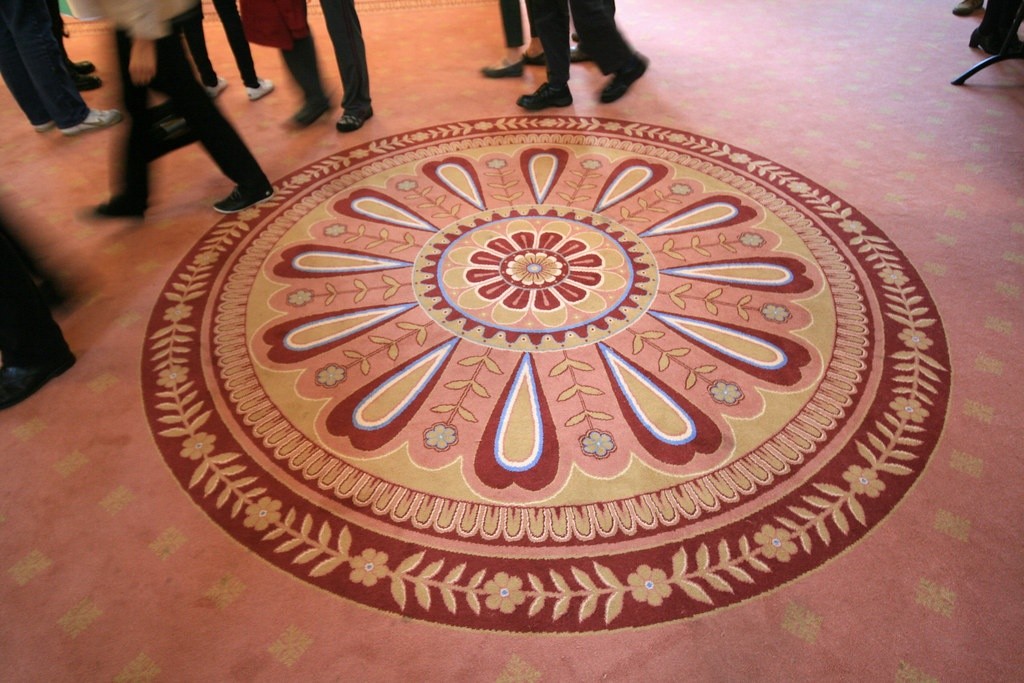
[1,1,375,411]
[953,0,1024,57]
[481,0,649,108]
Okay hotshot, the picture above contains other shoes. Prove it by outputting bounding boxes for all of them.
[74,61,96,73]
[296,97,331,124]
[334,106,372,133]
[570,46,588,62]
[952,0,984,15]
[480,59,524,77]
[521,50,547,64]
[570,32,579,39]
[76,73,101,92]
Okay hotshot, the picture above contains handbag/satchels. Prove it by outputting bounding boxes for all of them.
[146,97,199,162]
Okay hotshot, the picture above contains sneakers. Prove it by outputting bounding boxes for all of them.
[60,107,122,135]
[600,53,648,104]
[34,121,56,133]
[204,76,226,98]
[96,202,145,221]
[246,78,274,101]
[516,82,573,110]
[213,184,274,214]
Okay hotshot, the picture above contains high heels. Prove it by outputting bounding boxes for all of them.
[970,27,1024,56]
